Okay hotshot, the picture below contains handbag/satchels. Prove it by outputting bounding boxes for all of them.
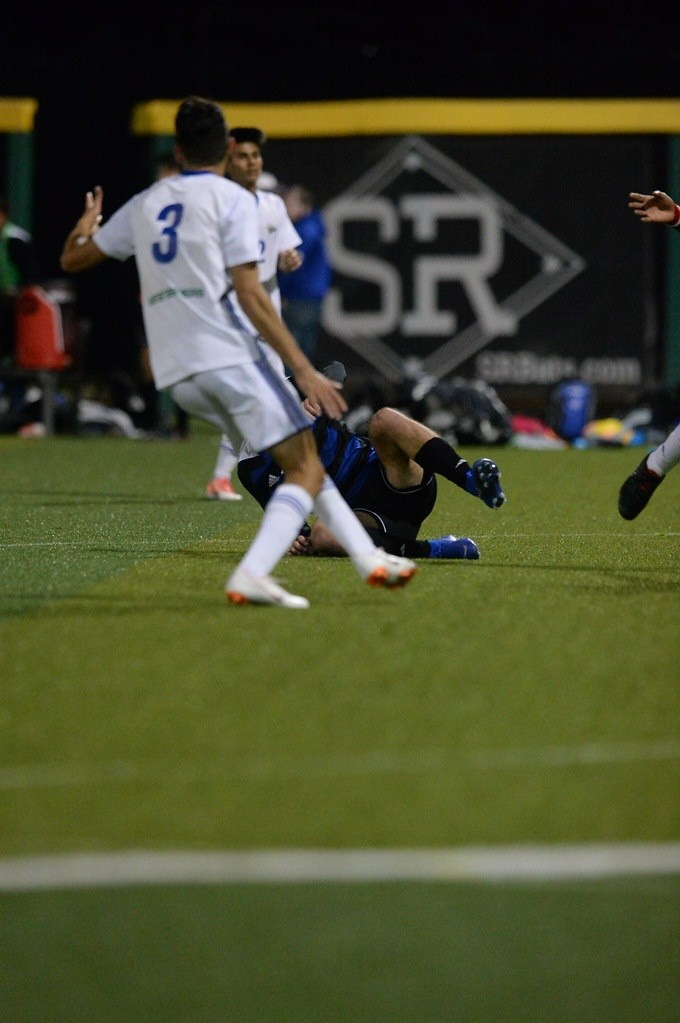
[407,369,651,450]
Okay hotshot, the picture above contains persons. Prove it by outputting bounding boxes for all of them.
[157,128,332,504]
[62,95,417,611]
[0,204,48,439]
[617,190,680,522]
[237,361,507,562]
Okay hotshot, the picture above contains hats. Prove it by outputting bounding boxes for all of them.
[229,128,262,145]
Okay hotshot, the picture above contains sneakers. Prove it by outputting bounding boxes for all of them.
[352,547,417,589]
[618,450,666,520]
[223,570,309,609]
[206,479,243,501]
[465,458,505,510]
[426,535,480,559]
